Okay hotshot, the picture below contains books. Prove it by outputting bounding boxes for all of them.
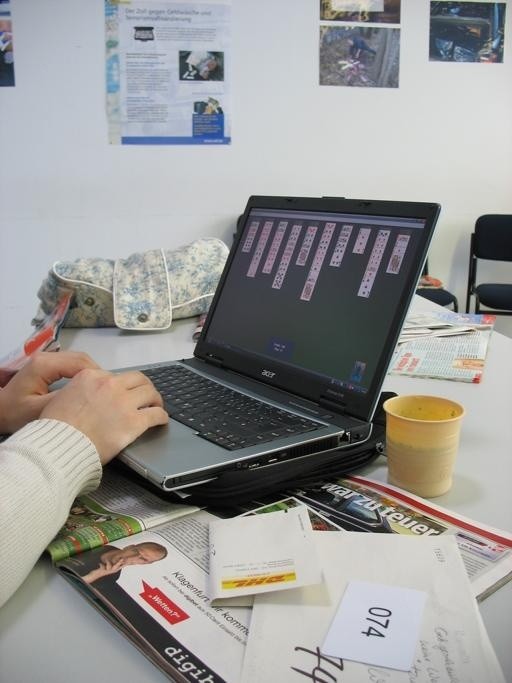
[44,449,511,682]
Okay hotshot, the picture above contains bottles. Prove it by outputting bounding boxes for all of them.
[491,26,503,53]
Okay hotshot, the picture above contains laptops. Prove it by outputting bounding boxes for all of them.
[49,196,441,495]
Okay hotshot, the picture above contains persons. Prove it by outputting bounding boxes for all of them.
[345,37,376,57]
[58,541,168,589]
[0,351,168,607]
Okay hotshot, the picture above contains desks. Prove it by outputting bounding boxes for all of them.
[1,294,512,683]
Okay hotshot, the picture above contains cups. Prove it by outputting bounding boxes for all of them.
[383,392,468,499]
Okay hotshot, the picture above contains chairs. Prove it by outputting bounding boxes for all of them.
[416,258,458,312]
[466,214,512,314]
